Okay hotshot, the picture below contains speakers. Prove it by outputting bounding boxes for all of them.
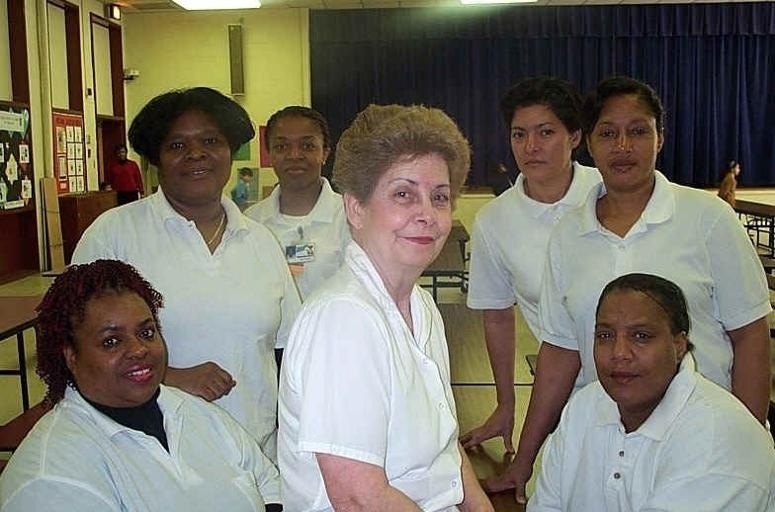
[227,24,245,97]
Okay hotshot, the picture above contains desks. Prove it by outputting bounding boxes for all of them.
[1,287,47,419]
[732,190,775,255]
[0,395,53,462]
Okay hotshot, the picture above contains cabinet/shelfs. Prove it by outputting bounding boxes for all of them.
[61,187,120,266]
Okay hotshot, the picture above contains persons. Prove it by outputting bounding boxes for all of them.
[716,160,740,212]
[107,144,144,205]
[521,272,775,512]
[457,77,604,455]
[1,258,283,512]
[241,105,353,302]
[68,84,303,462]
[276,101,497,512]
[474,74,773,507]
[229,166,253,212]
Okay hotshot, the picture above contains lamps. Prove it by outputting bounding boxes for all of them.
[121,68,140,83]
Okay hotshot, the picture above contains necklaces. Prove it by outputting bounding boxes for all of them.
[207,212,226,247]
